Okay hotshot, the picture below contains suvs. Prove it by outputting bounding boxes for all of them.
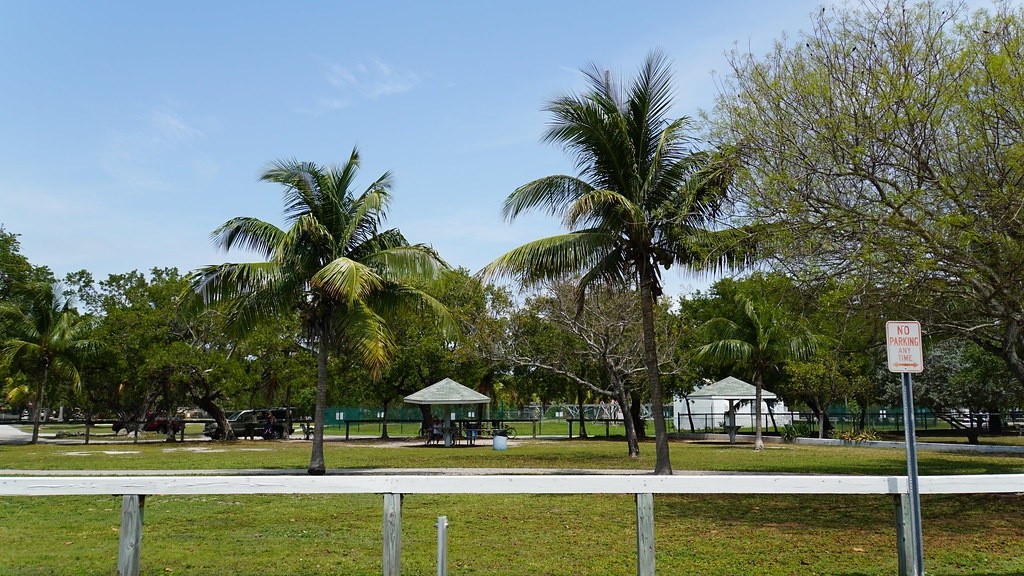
[202,407,296,441]
[112,409,186,436]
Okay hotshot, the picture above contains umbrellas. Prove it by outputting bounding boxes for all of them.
[403,377,490,446]
[687,376,777,443]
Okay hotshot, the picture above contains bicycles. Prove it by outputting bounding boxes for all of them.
[489,424,518,439]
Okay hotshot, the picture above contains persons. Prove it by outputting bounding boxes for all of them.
[427,416,442,447]
[243,413,254,440]
[264,411,276,434]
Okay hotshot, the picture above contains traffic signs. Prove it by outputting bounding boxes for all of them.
[886,320,925,374]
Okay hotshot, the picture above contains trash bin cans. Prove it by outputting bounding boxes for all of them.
[492,429,507,450]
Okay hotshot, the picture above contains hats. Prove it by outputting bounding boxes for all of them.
[265,412,272,415]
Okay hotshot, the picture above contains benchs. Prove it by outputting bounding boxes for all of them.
[426,429,475,447]
